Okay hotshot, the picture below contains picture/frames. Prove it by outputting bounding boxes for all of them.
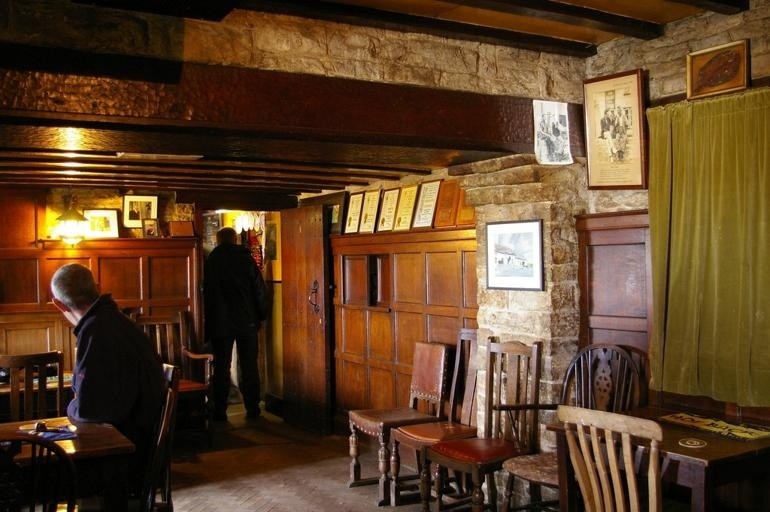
[433,179,460,229]
[455,178,480,230]
[411,179,443,229]
[683,38,754,100]
[580,66,649,191]
[376,186,401,232]
[82,206,120,240]
[393,184,418,231]
[359,187,382,234]
[121,194,158,228]
[483,219,545,293]
[346,193,365,233]
[142,218,159,237]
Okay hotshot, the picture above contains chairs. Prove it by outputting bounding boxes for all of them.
[500,342,641,512]
[125,308,214,440]
[556,404,663,512]
[348,339,450,508]
[137,382,178,512]
[427,335,542,512]
[0,348,66,424]
[0,434,76,512]
[390,325,493,510]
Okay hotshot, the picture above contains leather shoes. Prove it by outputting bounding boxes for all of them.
[248,406,261,417]
[214,409,226,422]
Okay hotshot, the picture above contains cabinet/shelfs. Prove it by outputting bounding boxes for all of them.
[0,235,201,372]
[334,224,481,433]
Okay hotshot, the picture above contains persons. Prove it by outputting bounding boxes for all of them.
[50,263,165,442]
[547,126,564,159]
[203,226,269,421]
[613,106,630,162]
[538,114,555,162]
[598,108,618,164]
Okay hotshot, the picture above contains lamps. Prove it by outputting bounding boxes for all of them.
[234,213,256,251]
[55,196,89,254]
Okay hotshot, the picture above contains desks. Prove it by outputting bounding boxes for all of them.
[0,414,133,512]
[544,399,770,512]
[0,365,76,405]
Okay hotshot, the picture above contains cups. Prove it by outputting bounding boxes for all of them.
[35,422,46,431]
